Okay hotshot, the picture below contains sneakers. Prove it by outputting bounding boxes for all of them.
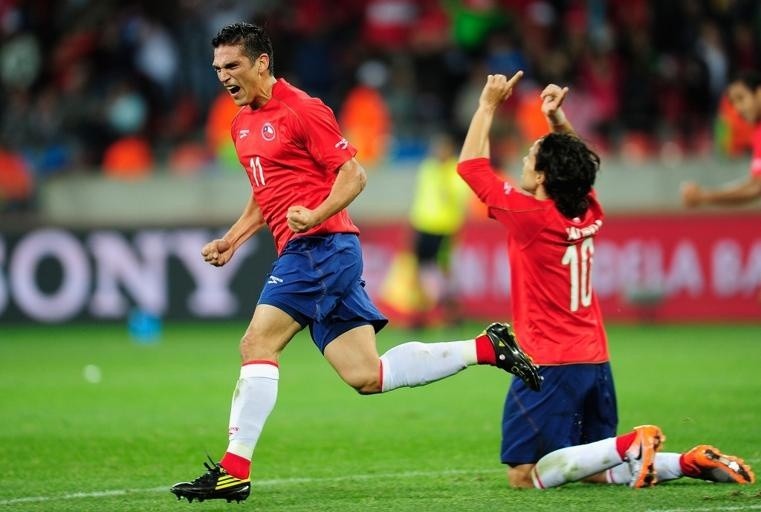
[487,321,544,393]
[169,463,250,504]
[685,444,755,484]
[624,424,665,487]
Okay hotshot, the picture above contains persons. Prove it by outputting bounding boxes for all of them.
[168,22,545,503]
[409,131,474,331]
[2,1,761,210]
[455,66,756,490]
